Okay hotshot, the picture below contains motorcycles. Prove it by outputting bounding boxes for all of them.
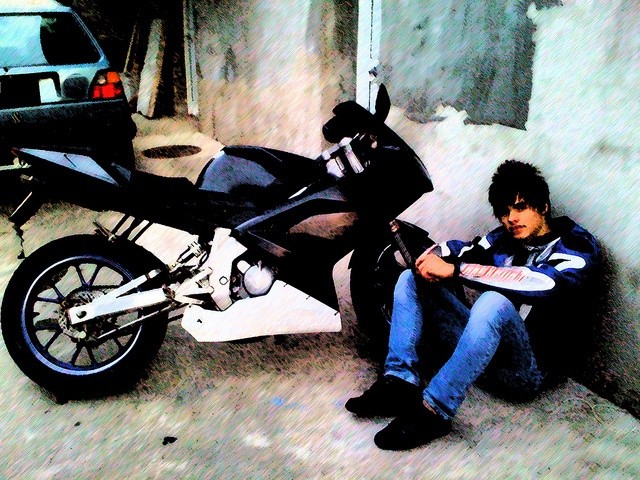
[0,83,439,402]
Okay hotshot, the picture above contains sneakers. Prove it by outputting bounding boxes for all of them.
[345,375,422,417]
[374,400,453,452]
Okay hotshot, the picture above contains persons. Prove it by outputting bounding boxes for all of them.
[345,159,600,450]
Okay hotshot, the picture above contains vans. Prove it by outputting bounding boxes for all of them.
[0,0,138,206]
